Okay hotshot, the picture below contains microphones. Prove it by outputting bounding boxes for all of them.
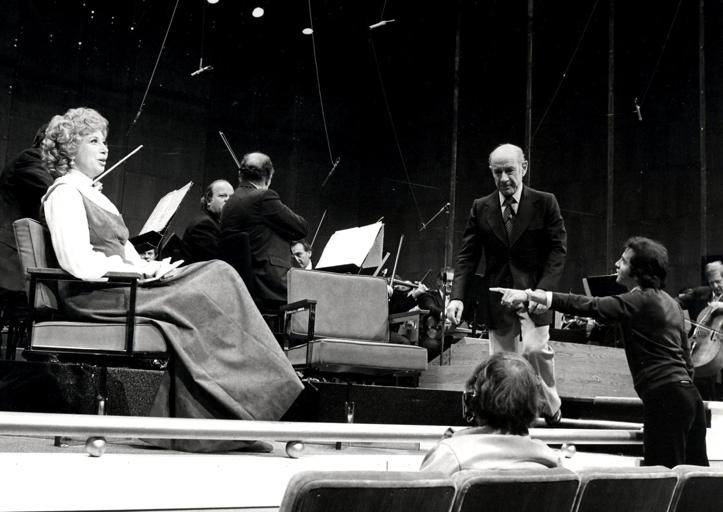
[443,201,451,210]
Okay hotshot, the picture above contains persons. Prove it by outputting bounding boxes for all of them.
[2,122,57,308]
[497,236,709,469]
[449,141,569,425]
[290,237,315,270]
[369,258,478,357]
[675,258,723,333]
[183,178,234,260]
[40,105,306,450]
[220,150,309,311]
[421,353,557,476]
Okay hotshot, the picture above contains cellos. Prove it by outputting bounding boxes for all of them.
[685,291,723,378]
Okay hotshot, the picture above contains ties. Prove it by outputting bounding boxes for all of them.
[503,198,516,236]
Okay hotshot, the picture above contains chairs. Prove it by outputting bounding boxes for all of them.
[673,461,723,511]
[276,267,428,449]
[4,217,177,450]
[451,466,582,511]
[569,464,680,512]
[277,468,454,510]
